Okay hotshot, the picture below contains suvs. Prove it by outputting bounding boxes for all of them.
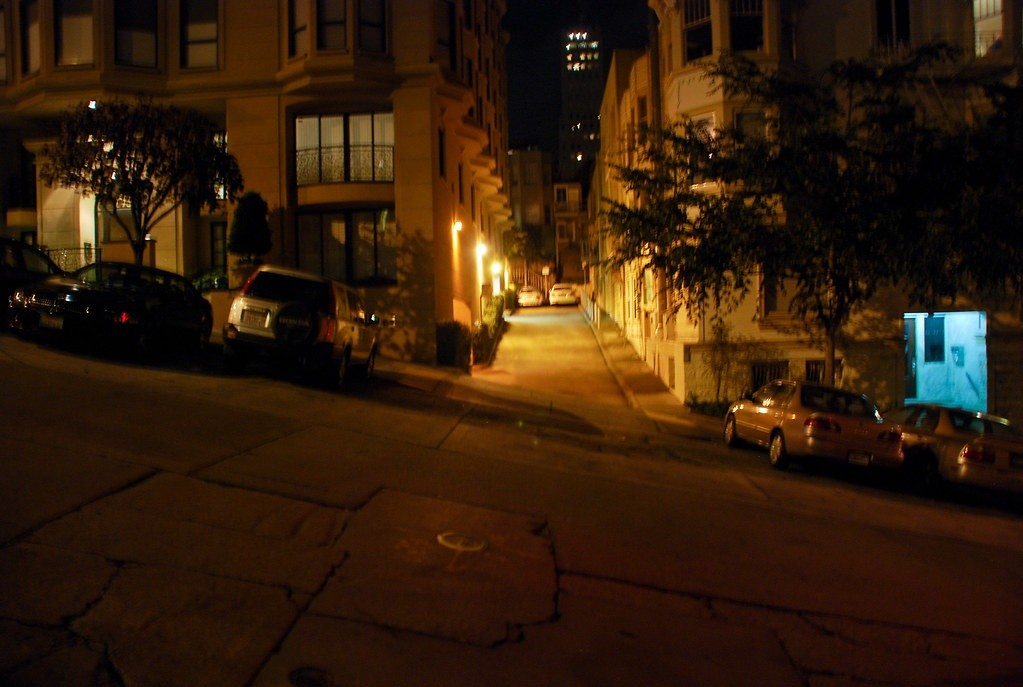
[222,263,381,389]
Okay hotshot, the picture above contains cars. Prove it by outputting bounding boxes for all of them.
[723,379,903,484]
[880,404,1023,504]
[7,262,213,364]
[516,288,543,306]
[549,283,576,306]
